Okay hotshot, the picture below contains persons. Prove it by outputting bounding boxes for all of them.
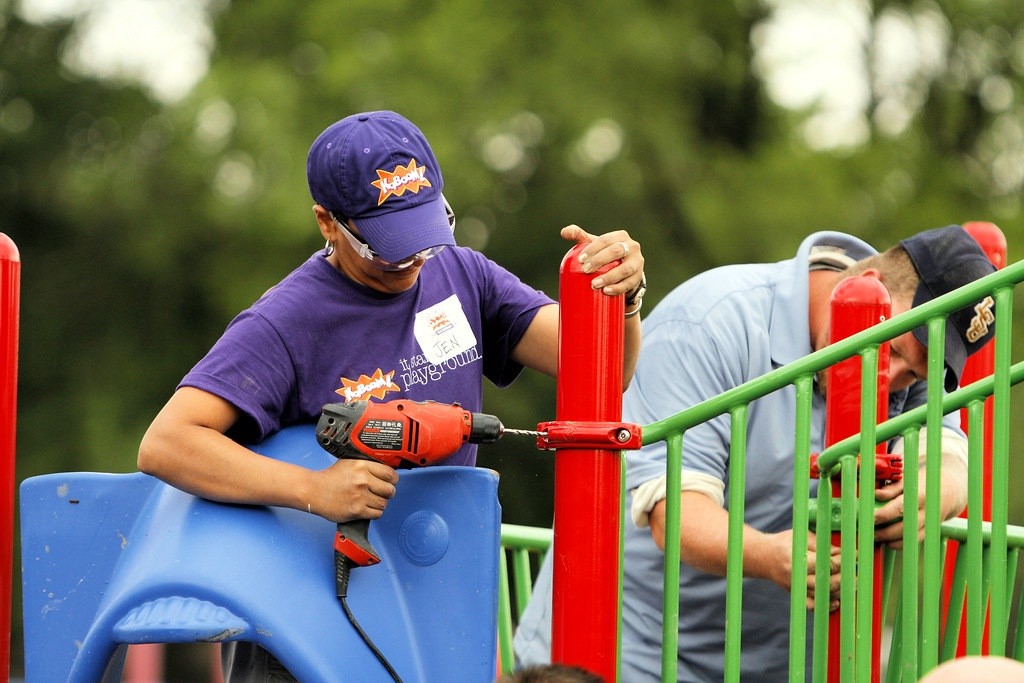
[512,223,1007,682]
[137,108,647,525]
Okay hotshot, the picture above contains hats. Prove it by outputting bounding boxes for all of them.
[304,109,457,264]
[899,223,998,395]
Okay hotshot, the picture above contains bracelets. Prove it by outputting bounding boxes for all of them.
[623,272,647,319]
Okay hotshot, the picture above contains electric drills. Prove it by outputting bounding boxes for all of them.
[313,397,549,567]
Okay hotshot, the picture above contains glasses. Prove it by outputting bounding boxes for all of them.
[327,191,456,272]
